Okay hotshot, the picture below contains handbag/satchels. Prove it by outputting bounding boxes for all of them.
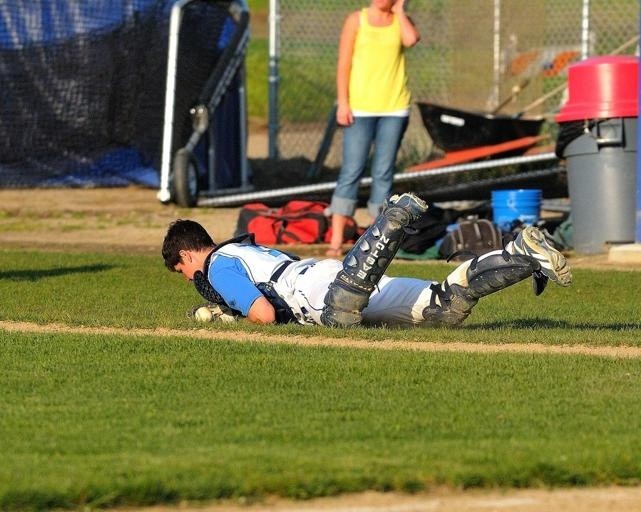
[394,102,446,174]
[437,216,502,261]
[233,200,359,245]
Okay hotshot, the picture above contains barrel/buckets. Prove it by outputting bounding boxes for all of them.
[489,189,541,235]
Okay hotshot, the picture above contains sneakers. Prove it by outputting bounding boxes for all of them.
[512,227,572,288]
[389,192,428,220]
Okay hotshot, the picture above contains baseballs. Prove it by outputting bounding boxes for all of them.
[195,307,212,323]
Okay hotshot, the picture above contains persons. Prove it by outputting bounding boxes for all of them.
[162,192,573,329]
[326,0,421,255]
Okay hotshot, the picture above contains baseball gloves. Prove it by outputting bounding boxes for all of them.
[192,301,238,323]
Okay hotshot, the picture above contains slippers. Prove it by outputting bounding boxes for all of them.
[326,247,344,256]
